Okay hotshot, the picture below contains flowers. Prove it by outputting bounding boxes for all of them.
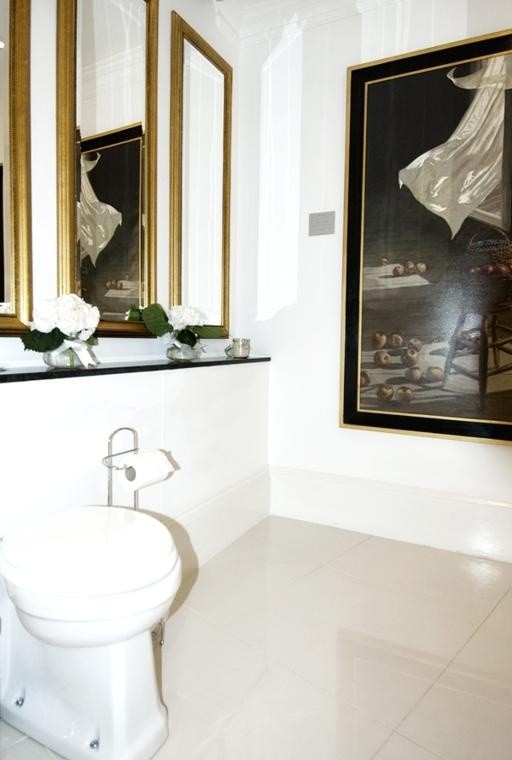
[19,285,99,366]
[131,295,206,352]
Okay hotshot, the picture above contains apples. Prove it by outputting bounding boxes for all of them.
[387,333,404,346]
[405,367,421,382]
[380,257,427,276]
[372,333,387,349]
[378,385,393,401]
[470,264,512,275]
[426,366,441,379]
[402,349,416,364]
[374,350,390,365]
[396,387,412,404]
[407,337,422,352]
[360,372,370,386]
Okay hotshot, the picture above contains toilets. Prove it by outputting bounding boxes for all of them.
[0,504,181,760]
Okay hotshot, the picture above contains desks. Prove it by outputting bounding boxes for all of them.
[361,263,434,303]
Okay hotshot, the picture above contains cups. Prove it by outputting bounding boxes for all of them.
[224,337,250,359]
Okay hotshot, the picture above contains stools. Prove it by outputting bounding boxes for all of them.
[429,285,510,415]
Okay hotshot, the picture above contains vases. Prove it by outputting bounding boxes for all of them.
[38,340,97,371]
[162,337,201,365]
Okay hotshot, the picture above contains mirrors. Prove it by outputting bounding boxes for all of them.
[163,6,237,341]
[50,0,164,339]
[0,0,38,339]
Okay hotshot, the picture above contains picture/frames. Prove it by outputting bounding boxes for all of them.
[339,26,512,445]
[75,121,149,325]
